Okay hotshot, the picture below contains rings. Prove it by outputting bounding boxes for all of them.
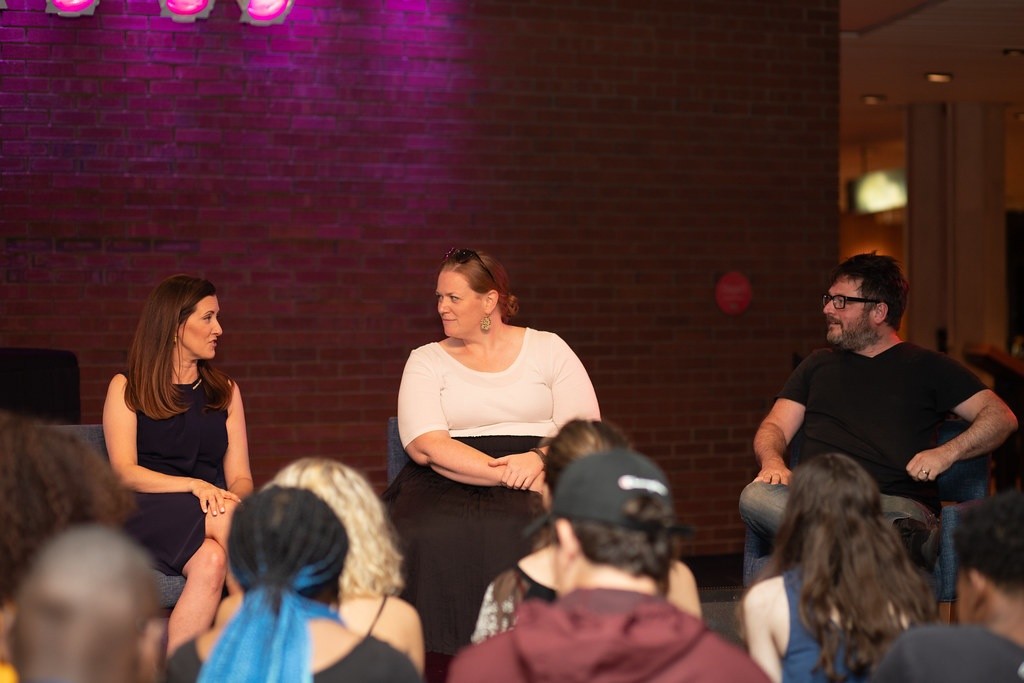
[920,470,929,475]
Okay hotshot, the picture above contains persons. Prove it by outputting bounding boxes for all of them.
[879,489,1024,683]
[448,449,774,683]
[742,454,938,683]
[738,253,1018,575]
[379,245,602,658]
[472,416,702,645]
[4,522,164,683]
[102,275,254,658]
[0,423,126,683]
[165,483,421,683]
[213,456,426,674]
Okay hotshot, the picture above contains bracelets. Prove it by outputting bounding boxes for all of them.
[531,448,546,471]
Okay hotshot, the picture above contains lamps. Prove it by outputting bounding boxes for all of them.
[158,0,216,23]
[237,0,294,27]
[45,0,100,17]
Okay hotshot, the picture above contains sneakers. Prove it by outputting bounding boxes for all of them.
[896,520,939,574]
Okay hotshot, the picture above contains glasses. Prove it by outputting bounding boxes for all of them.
[445,248,496,283]
[822,294,883,308]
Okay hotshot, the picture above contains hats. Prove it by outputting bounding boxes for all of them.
[521,451,676,538]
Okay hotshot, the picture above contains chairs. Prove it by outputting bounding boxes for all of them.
[743,418,986,626]
[33,424,186,608]
